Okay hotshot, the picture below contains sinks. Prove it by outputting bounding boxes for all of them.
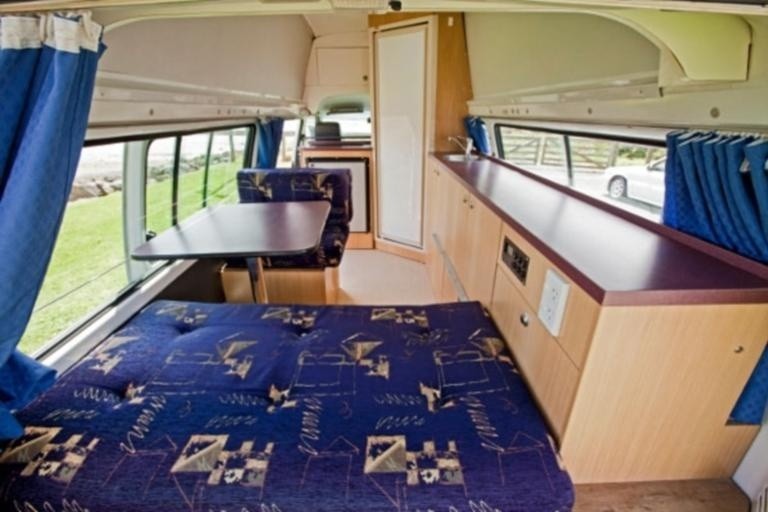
[443,154,479,162]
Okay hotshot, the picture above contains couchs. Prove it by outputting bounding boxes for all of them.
[219,168,353,305]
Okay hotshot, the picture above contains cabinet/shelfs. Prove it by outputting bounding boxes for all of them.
[423,163,503,314]
[488,223,605,455]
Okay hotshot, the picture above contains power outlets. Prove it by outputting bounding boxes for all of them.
[537,268,570,337]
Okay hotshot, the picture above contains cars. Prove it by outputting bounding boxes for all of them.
[601,155,667,209]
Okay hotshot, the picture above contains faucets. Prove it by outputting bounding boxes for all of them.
[448,136,473,158]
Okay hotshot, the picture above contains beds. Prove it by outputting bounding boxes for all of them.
[0,298,576,512]
